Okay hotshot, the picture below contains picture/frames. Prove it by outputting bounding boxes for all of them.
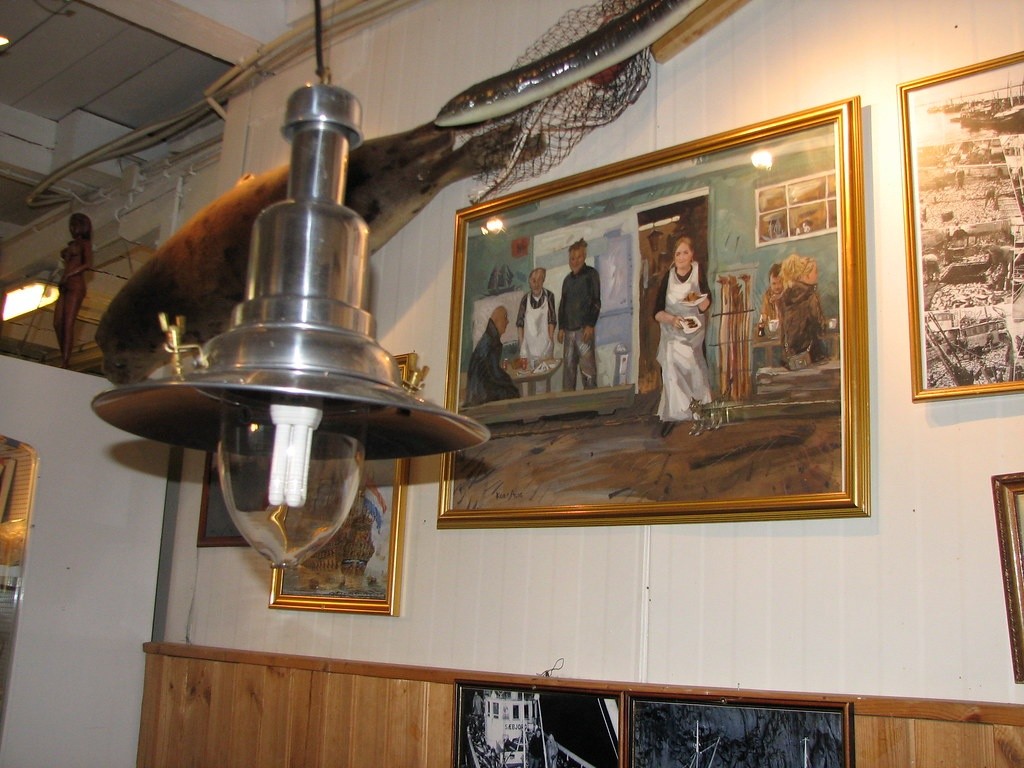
[438,94,873,530]
[991,472,1024,685]
[268,353,418,616]
[195,450,249,549]
[622,691,855,768]
[896,50,1024,402]
[450,678,625,768]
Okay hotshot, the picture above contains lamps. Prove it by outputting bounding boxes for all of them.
[91,0,489,569]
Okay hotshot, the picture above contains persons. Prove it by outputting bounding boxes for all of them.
[52,212,92,367]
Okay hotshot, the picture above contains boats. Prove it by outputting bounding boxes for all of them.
[465,691,595,768]
[927,74,1024,129]
